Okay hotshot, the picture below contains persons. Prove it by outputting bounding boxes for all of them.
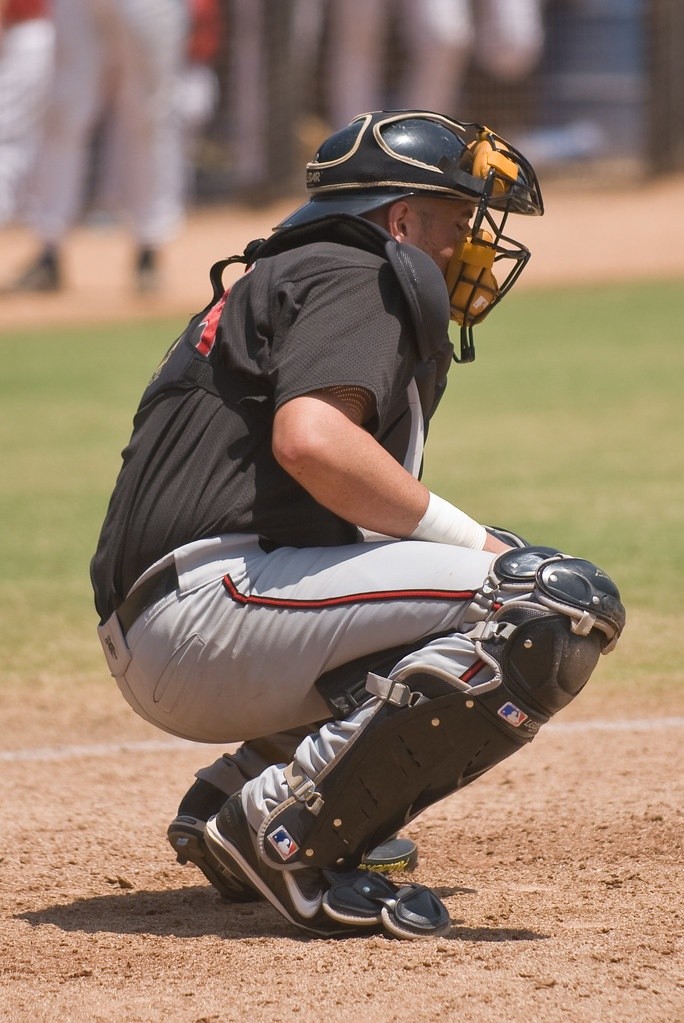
[1,0,545,293]
[90,110,626,940]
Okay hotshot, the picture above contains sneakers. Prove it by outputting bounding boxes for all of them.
[204,788,384,939]
[167,778,259,902]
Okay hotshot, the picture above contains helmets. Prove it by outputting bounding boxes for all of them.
[272,118,468,232]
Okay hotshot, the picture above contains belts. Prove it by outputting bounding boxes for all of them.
[116,539,283,637]
